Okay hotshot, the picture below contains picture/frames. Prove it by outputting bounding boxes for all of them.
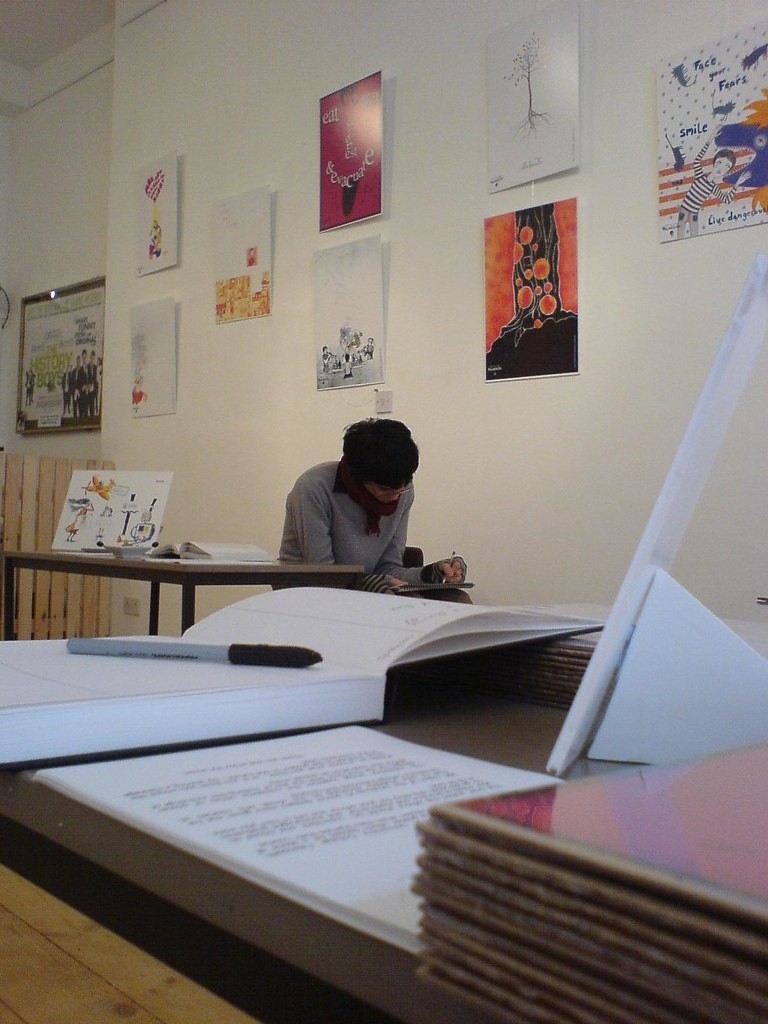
[13,274,106,436]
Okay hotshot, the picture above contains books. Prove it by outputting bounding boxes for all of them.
[407,738,767,1024]
[151,542,275,562]
[400,631,603,709]
[0,587,606,764]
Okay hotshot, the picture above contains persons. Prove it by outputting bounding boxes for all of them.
[272,419,474,606]
[62,349,98,418]
[25,370,36,408]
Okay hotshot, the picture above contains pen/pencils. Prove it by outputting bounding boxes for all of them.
[442,551,456,583]
[66,637,323,669]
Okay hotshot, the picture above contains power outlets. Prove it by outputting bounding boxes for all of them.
[123,595,140,616]
[374,389,393,414]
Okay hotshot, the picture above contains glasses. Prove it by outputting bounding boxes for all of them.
[368,484,414,504]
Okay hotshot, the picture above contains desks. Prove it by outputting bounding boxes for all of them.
[0,671,637,1024]
[0,550,365,641]
